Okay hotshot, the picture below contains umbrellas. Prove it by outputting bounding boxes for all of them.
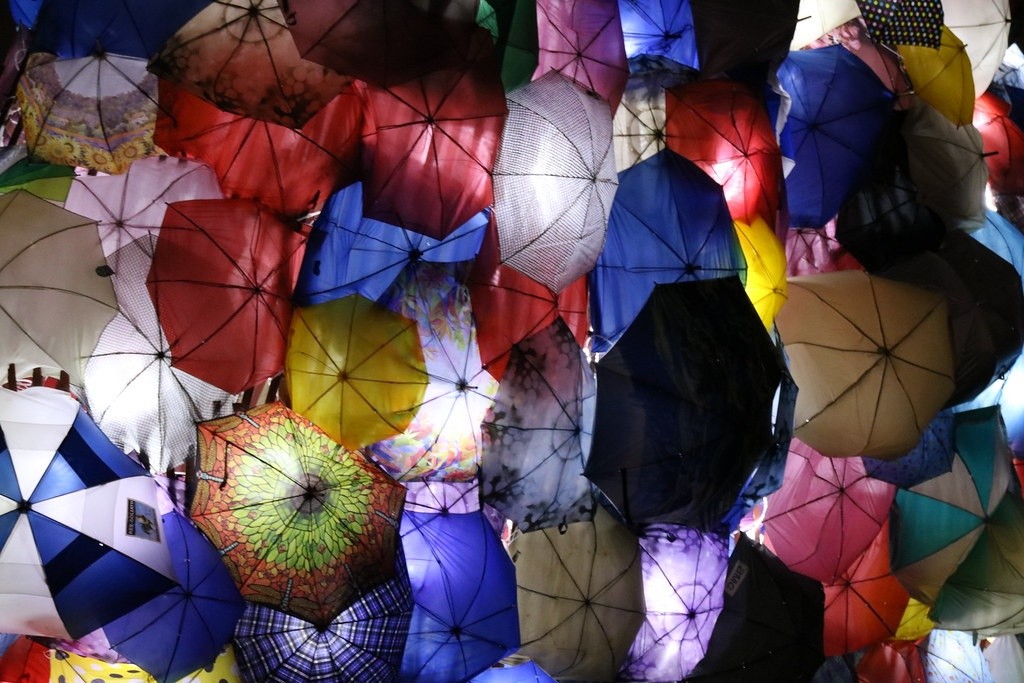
[489,64,622,292]
[0,0,1024,683]
